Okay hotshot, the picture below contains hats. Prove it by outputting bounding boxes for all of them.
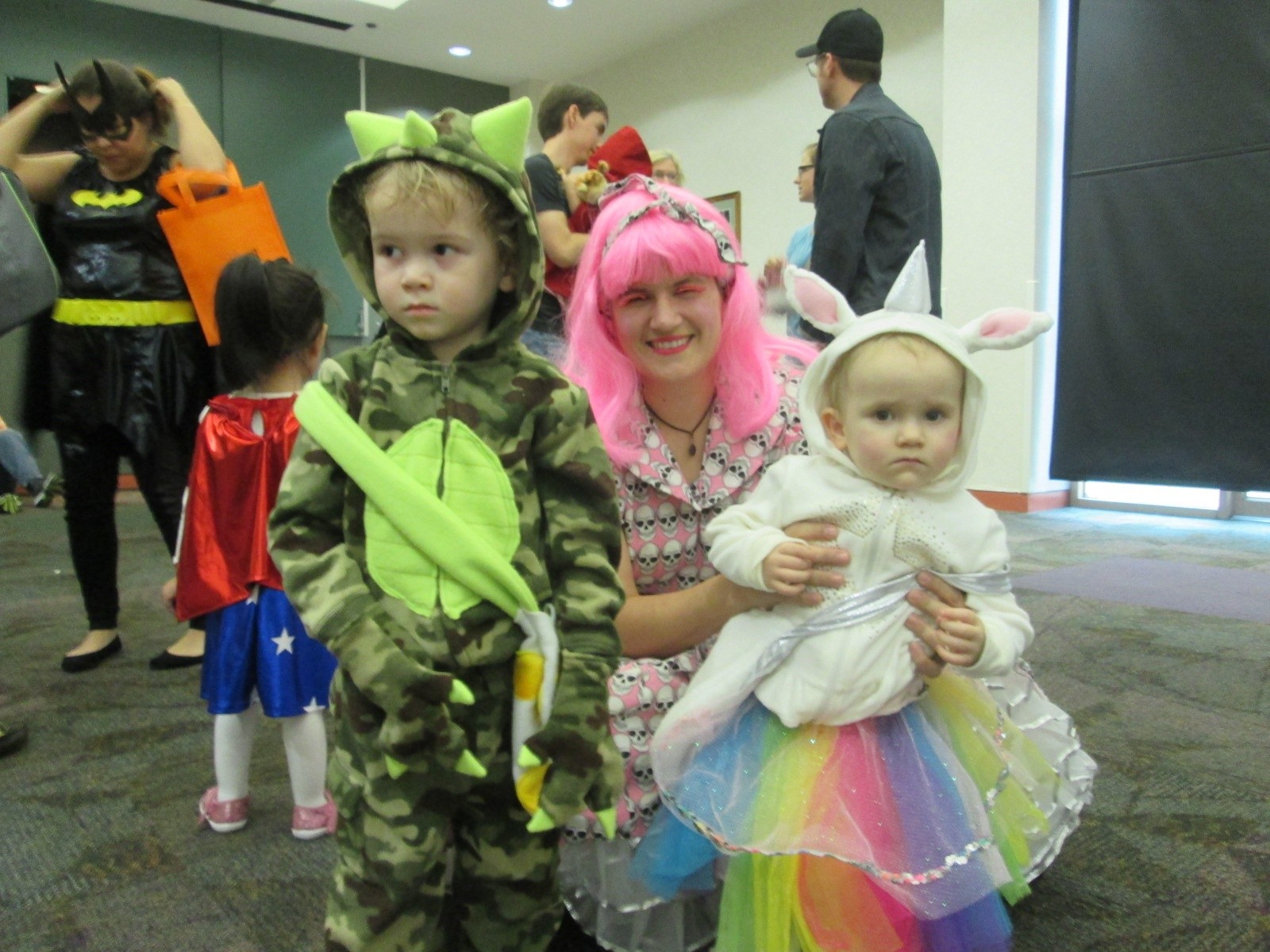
[588,125,653,182]
[795,8,884,62]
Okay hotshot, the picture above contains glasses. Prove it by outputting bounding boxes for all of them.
[798,165,816,171]
[806,55,825,78]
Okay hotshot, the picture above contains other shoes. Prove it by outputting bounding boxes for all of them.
[149,648,205,671]
[62,634,122,673]
[199,785,250,833]
[291,790,339,841]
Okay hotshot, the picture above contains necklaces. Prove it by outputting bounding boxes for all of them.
[641,387,717,457]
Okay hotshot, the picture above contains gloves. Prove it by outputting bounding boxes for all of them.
[312,586,487,781]
[517,648,626,843]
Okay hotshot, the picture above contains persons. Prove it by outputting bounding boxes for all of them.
[0,416,58,508]
[518,82,683,363]
[176,250,335,840]
[0,62,245,670]
[700,263,1054,952]
[757,7,941,344]
[267,95,626,952]
[558,172,971,952]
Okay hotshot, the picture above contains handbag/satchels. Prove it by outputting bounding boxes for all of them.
[155,156,294,348]
